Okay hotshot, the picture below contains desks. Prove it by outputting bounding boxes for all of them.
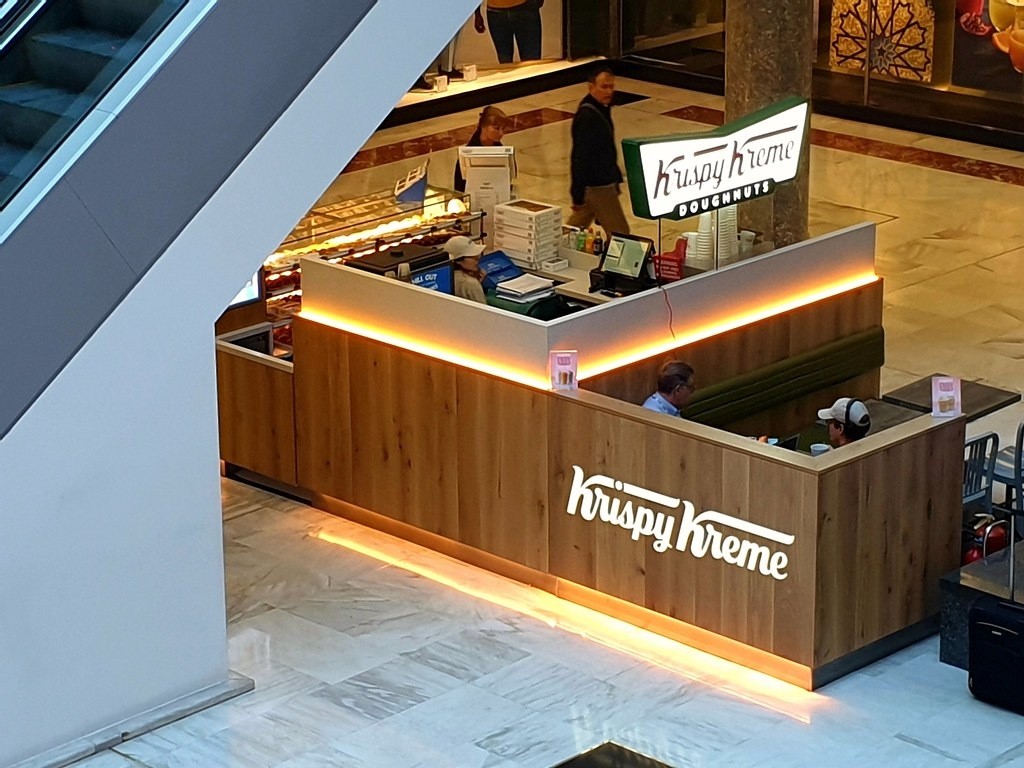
[884,371,1022,424]
[817,398,925,437]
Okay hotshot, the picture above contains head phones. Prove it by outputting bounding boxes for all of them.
[844,397,867,439]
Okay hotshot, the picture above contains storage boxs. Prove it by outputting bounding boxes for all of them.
[478,251,518,287]
[492,198,569,272]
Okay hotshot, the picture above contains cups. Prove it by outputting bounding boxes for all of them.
[397,263,411,285]
[944,395,956,410]
[939,398,950,412]
[811,443,828,456]
[682,203,755,260]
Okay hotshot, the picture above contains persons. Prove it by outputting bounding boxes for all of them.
[454,105,506,191]
[473,0,544,64]
[818,396,871,444]
[565,72,630,239]
[443,235,487,304]
[644,360,695,416]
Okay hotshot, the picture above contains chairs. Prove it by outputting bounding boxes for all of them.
[981,420,1024,523]
[963,432,999,548]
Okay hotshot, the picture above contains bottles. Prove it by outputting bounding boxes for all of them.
[575,225,586,252]
[569,228,577,251]
[586,228,595,254]
[592,231,603,255]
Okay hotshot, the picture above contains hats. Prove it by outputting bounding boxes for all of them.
[442,236,487,260]
[817,397,871,427]
[479,106,515,128]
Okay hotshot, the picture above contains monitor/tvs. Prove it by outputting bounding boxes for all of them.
[597,230,654,282]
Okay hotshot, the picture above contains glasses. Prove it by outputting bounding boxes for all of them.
[674,381,696,393]
